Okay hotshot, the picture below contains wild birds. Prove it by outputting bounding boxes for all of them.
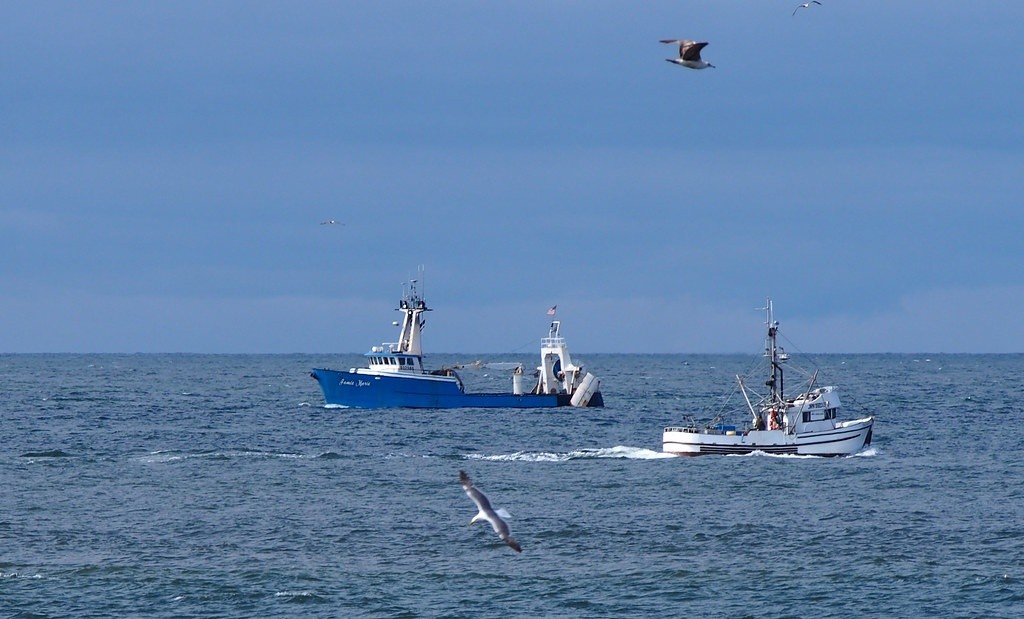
[459,466,525,552]
[659,38,717,70]
[791,0,822,16]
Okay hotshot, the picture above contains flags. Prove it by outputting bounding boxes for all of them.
[546,304,557,315]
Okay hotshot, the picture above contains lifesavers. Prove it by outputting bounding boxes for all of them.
[769,417,779,429]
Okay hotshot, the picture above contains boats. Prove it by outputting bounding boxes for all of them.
[310,264,605,409]
[662,296,875,457]
[452,359,525,371]
